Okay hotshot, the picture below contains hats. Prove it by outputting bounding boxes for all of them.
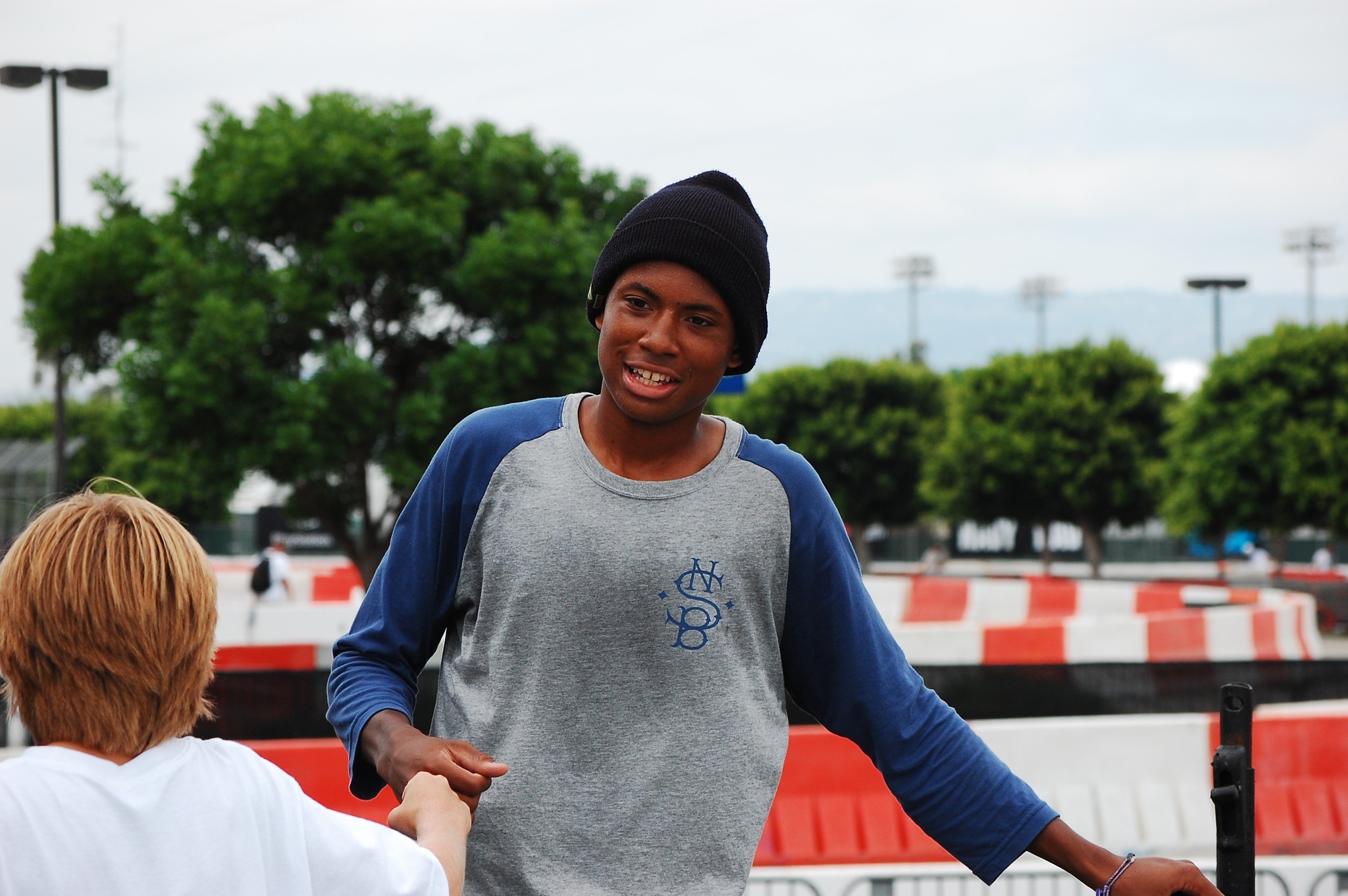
[586,170,770,377]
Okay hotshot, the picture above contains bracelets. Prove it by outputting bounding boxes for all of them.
[1096,852,1136,895]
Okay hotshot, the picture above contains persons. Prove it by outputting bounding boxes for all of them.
[243,538,299,618]
[326,172,1225,895]
[1,477,472,896]
